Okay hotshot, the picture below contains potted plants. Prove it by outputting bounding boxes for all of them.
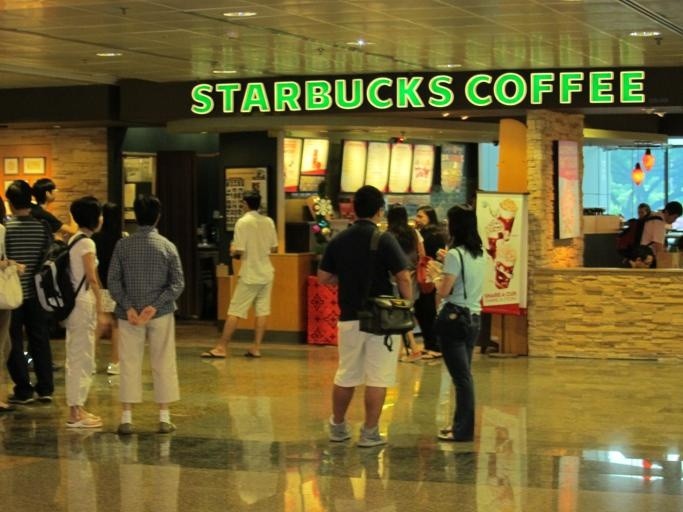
[2,156,46,194]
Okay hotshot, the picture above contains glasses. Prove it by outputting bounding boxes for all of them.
[641,148,656,172]
[632,162,644,187]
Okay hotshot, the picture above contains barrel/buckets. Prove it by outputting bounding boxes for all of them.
[245,350,261,357]
[199,351,224,358]
[422,352,443,359]
[64,412,103,428]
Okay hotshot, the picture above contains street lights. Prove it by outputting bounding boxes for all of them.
[359,295,414,336]
[436,302,471,340]
[0,265,24,310]
[417,255,435,293]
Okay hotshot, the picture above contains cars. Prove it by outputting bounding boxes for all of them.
[485,205,515,288]
[425,258,443,284]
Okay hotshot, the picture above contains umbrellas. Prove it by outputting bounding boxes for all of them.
[437,427,456,441]
[0,404,16,411]
[118,423,134,434]
[160,422,175,433]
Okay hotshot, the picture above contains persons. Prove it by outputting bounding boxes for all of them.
[416,206,445,358]
[119,434,180,512]
[202,190,278,358]
[387,364,446,512]
[63,428,98,512]
[318,185,415,447]
[106,195,185,435]
[426,202,497,442]
[616,202,683,268]
[331,442,381,512]
[4,403,59,512]
[385,203,425,363]
[214,359,280,512]
[0,179,128,429]
[449,443,478,512]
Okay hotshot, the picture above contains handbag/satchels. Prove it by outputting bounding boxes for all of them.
[431,277,443,284]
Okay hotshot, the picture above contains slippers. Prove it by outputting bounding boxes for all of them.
[33,234,89,321]
[618,212,662,246]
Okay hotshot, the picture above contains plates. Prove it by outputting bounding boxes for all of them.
[214,251,317,345]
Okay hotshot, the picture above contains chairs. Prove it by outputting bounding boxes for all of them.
[93,358,105,372]
[7,394,35,405]
[402,351,421,362]
[328,422,351,442]
[107,361,120,375]
[38,395,52,402]
[355,425,385,447]
